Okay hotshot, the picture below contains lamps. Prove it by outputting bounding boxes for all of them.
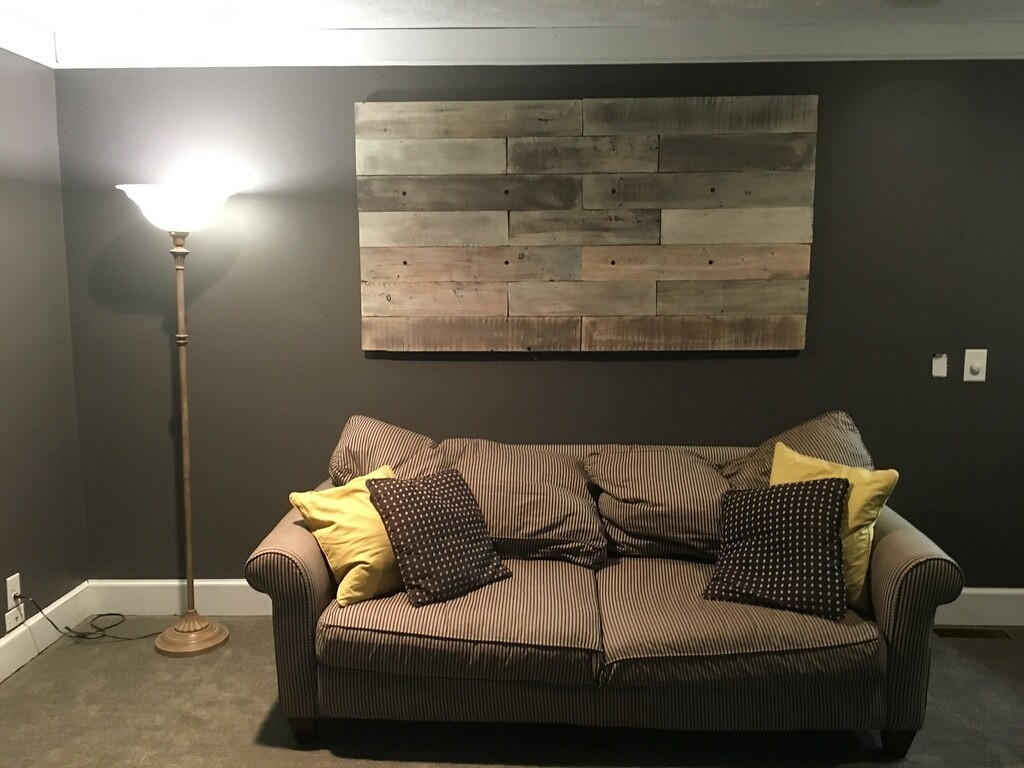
[113,179,230,658]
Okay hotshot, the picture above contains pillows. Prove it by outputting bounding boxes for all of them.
[769,441,899,604]
[365,469,512,607]
[288,465,403,607]
[702,475,850,620]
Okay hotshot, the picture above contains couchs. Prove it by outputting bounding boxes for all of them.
[241,409,964,763]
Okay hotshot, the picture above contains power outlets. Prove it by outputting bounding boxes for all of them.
[5,603,25,633]
[6,572,22,610]
